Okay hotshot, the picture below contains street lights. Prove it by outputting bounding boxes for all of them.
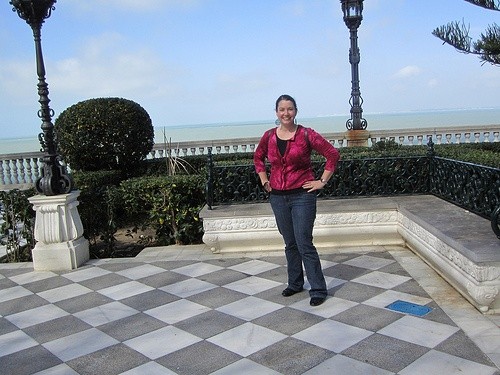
[9,0,89,273]
[340,0,371,147]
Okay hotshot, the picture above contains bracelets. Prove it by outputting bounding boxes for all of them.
[262,180,268,186]
[320,179,327,184]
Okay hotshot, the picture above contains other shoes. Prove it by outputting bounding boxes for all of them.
[282,286,303,296]
[310,297,325,306]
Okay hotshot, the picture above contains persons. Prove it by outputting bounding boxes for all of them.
[253,95,341,306]
